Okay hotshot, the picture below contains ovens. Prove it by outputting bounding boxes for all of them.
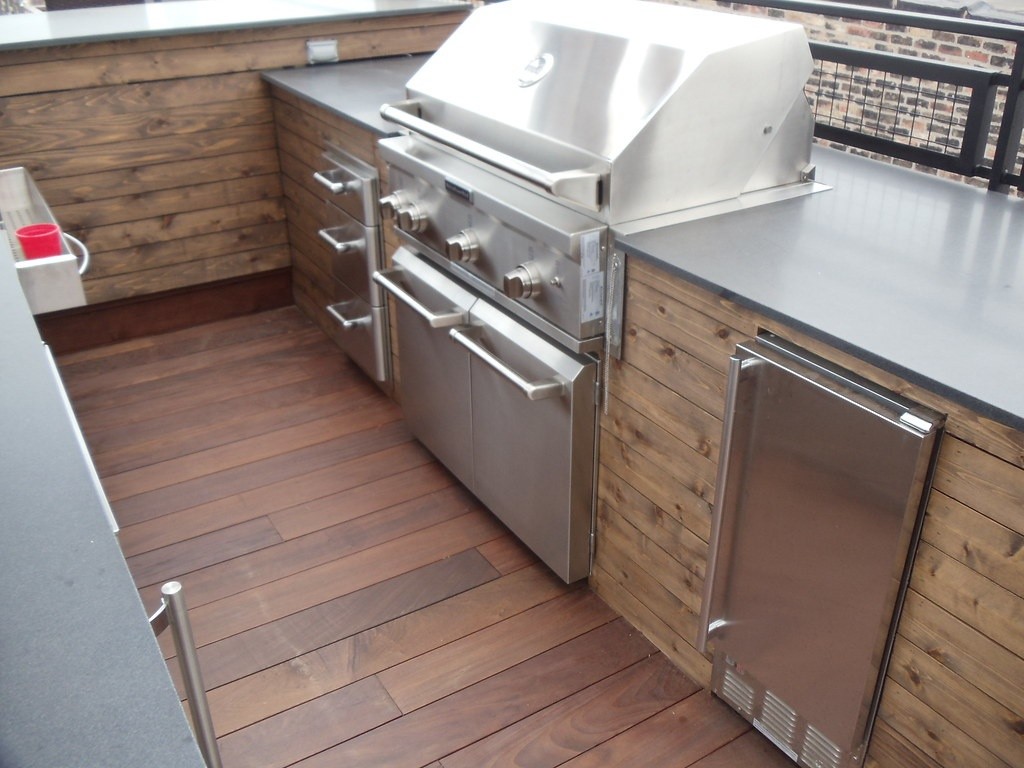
[371,246,600,584]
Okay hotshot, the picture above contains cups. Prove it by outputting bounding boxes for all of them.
[16,223,62,260]
